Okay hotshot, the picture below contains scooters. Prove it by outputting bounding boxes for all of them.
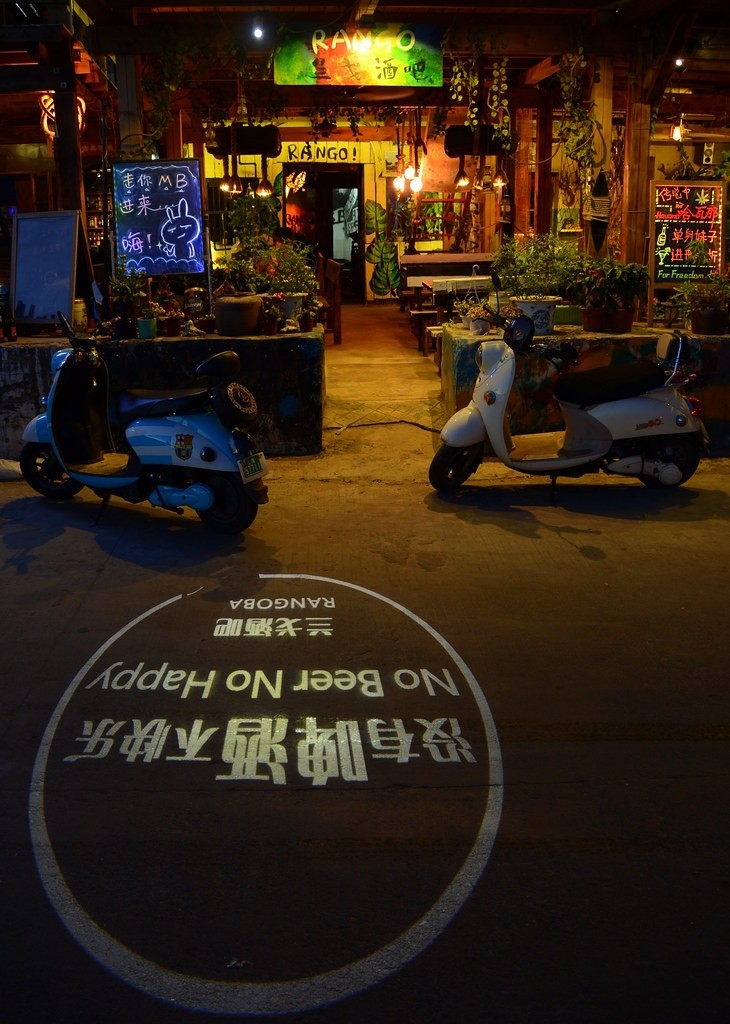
[19,312,270,533]
[428,269,714,493]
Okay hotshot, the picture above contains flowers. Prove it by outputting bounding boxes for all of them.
[142,301,166,319]
[566,252,651,309]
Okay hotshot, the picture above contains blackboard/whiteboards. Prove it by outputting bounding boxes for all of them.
[648,182,726,289]
[109,157,211,275]
[9,210,94,325]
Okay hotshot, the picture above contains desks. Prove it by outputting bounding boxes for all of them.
[407,276,538,357]
[400,252,496,273]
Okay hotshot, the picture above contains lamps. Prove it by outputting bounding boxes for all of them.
[456,57,510,187]
[214,71,273,199]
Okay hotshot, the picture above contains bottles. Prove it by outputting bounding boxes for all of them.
[8,318,17,341]
[0,316,4,343]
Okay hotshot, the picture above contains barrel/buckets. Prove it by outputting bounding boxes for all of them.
[72,298,88,331]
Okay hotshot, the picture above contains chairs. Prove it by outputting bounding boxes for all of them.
[301,252,341,346]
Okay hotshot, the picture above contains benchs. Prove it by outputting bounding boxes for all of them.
[403,288,475,352]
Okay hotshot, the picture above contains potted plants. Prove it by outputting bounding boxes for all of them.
[198,313,215,335]
[164,310,184,337]
[489,228,582,335]
[109,254,147,339]
[454,299,496,334]
[214,238,324,336]
[670,239,730,335]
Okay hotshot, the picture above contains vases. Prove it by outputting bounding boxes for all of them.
[578,304,637,333]
[137,317,156,340]
